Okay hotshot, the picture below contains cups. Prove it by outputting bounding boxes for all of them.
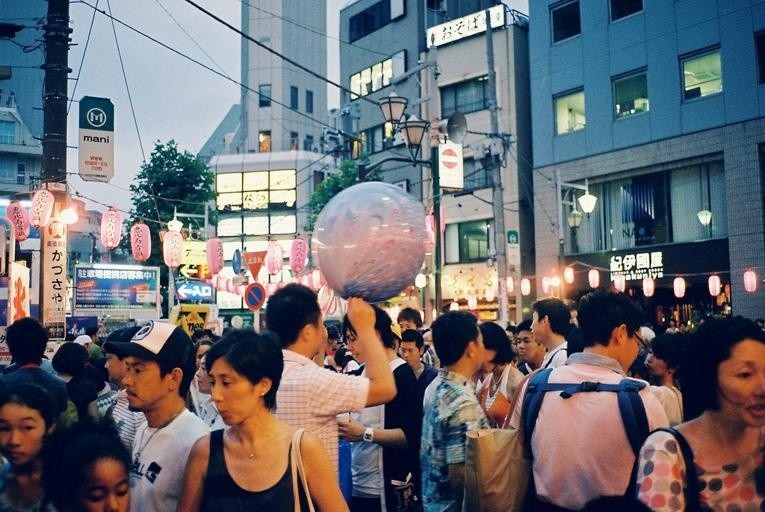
[632,331,647,353]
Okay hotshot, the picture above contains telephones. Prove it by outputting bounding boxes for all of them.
[174,281,213,301]
[197,265,211,280]
[174,281,213,300]
[179,264,198,278]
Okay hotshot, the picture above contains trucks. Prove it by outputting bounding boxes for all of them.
[315,182,427,301]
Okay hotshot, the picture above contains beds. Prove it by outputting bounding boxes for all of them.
[506,289,670,512]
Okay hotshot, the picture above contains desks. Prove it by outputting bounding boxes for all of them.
[378,85,441,318]
[552,169,598,302]
[378,81,444,330]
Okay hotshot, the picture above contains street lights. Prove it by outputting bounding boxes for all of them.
[463,368,546,512]
[577,425,712,512]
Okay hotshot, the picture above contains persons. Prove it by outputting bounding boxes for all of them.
[504,325,518,355]
[73,335,93,352]
[530,297,571,369]
[644,332,683,427]
[81,366,118,419]
[635,320,655,342]
[342,335,360,373]
[41,422,133,512]
[195,340,213,370]
[190,329,213,345]
[475,321,525,428]
[419,310,491,512]
[666,319,679,333]
[265,283,397,485]
[121,320,212,512]
[0,381,60,512]
[680,321,687,332]
[51,342,100,420]
[335,305,418,512]
[634,316,765,511]
[84,324,104,360]
[516,318,548,368]
[400,328,439,392]
[176,330,350,512]
[0,317,68,414]
[325,326,338,356]
[102,326,147,454]
[397,307,436,366]
[195,349,218,431]
[390,321,402,358]
[627,358,664,409]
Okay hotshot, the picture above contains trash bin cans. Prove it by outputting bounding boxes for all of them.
[363,427,374,442]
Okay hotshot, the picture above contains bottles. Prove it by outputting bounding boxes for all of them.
[103,320,194,371]
[73,335,92,346]
[390,320,402,340]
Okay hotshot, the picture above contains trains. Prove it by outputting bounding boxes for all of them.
[439,139,464,189]
[245,283,265,311]
[244,283,266,312]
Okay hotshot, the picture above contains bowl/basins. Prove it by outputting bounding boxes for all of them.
[226,428,255,458]
[135,409,178,458]
[492,364,508,391]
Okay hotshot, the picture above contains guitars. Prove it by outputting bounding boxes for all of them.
[614,274,625,292]
[468,295,477,310]
[266,240,283,275]
[485,287,494,301]
[709,275,721,296]
[29,189,54,229]
[521,278,530,296]
[564,268,574,283]
[542,276,549,294]
[506,277,514,292]
[130,222,151,262]
[588,270,600,288]
[673,277,685,298]
[162,231,183,268]
[290,237,307,273]
[6,199,29,241]
[643,278,654,296]
[744,270,756,292]
[449,302,459,311]
[206,236,224,275]
[100,209,122,250]
